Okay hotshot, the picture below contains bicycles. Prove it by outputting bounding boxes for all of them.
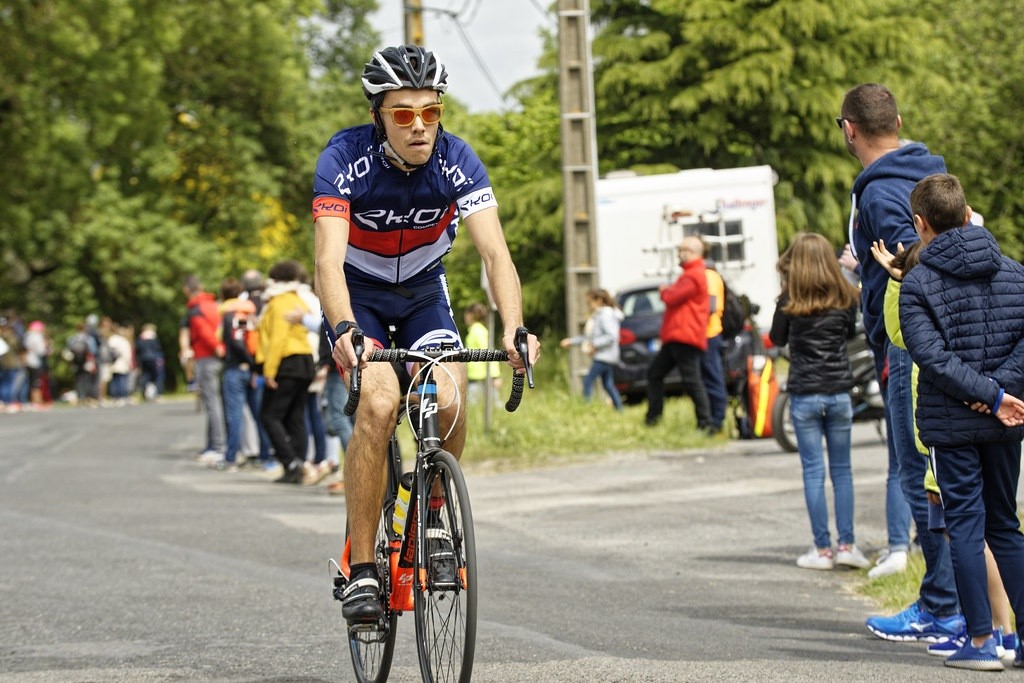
[328,325,534,683]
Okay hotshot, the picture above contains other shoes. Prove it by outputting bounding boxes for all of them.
[197,451,343,494]
[0,390,158,413]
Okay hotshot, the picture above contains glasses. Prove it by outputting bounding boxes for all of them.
[379,102,445,127]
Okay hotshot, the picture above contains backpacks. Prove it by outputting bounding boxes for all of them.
[734,353,779,440]
[705,267,746,337]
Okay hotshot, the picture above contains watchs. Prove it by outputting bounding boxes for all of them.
[334,320,360,341]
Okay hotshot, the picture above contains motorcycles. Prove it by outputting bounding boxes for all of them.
[771,315,886,452]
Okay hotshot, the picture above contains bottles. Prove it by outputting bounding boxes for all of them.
[392,473,414,534]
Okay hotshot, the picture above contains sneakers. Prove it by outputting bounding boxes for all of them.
[866,601,967,644]
[928,628,1017,660]
[943,637,1024,670]
[868,551,907,578]
[341,568,382,620]
[836,543,870,569]
[424,516,458,582]
[796,547,834,569]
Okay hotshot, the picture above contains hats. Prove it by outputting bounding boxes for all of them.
[267,262,298,282]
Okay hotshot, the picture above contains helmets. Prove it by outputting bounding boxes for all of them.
[360,45,448,101]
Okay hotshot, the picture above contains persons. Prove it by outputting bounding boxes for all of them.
[870,211,1016,661]
[0,314,164,414]
[769,232,872,570]
[559,287,625,413]
[177,261,355,494]
[839,139,923,581]
[463,302,504,410]
[312,44,542,620]
[698,241,726,435]
[898,172,1024,670]
[643,235,715,436]
[836,82,967,642]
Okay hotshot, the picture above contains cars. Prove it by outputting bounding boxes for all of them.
[610,283,762,405]
